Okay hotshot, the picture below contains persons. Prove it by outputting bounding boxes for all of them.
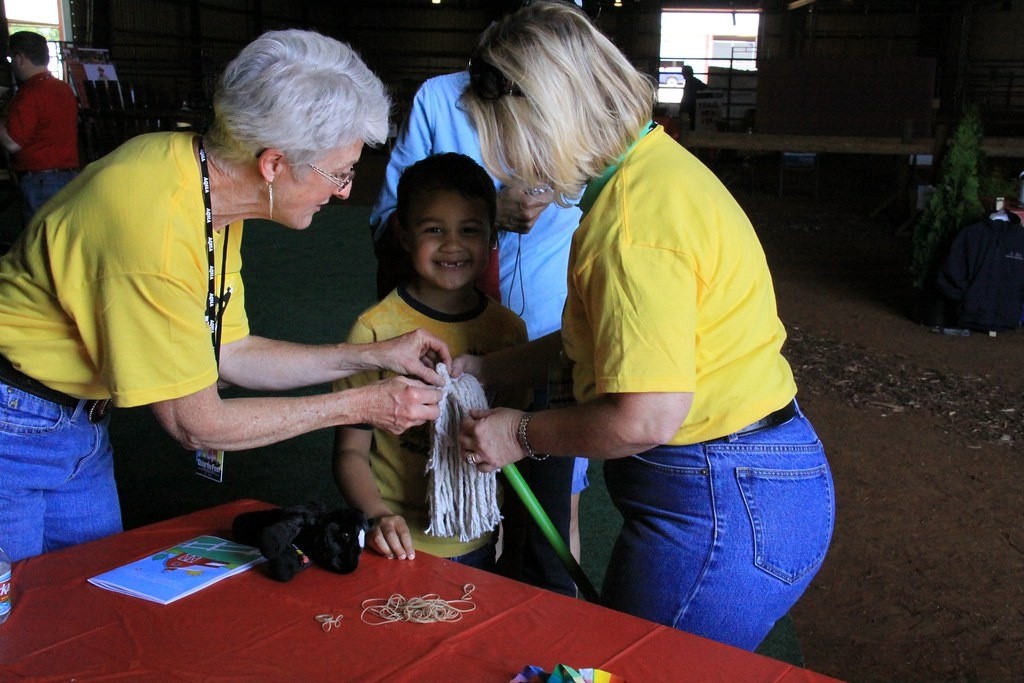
[331,152,535,572]
[372,70,589,598]
[0,32,79,225]
[0,29,453,564]
[450,0,836,652]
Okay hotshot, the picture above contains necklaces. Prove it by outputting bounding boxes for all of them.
[577,119,658,214]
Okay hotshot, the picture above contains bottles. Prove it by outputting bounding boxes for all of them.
[0,544,12,625]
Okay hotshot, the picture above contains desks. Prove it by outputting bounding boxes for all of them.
[3,495,839,683]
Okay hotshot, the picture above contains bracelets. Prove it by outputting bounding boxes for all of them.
[519,415,549,461]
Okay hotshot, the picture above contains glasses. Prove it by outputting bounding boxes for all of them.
[5,51,24,64]
[256,146,362,192]
[466,57,526,101]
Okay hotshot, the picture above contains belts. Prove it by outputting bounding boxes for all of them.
[735,399,797,436]
[0,353,115,424]
[18,168,80,175]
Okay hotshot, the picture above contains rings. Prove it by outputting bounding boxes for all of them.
[467,453,475,465]
